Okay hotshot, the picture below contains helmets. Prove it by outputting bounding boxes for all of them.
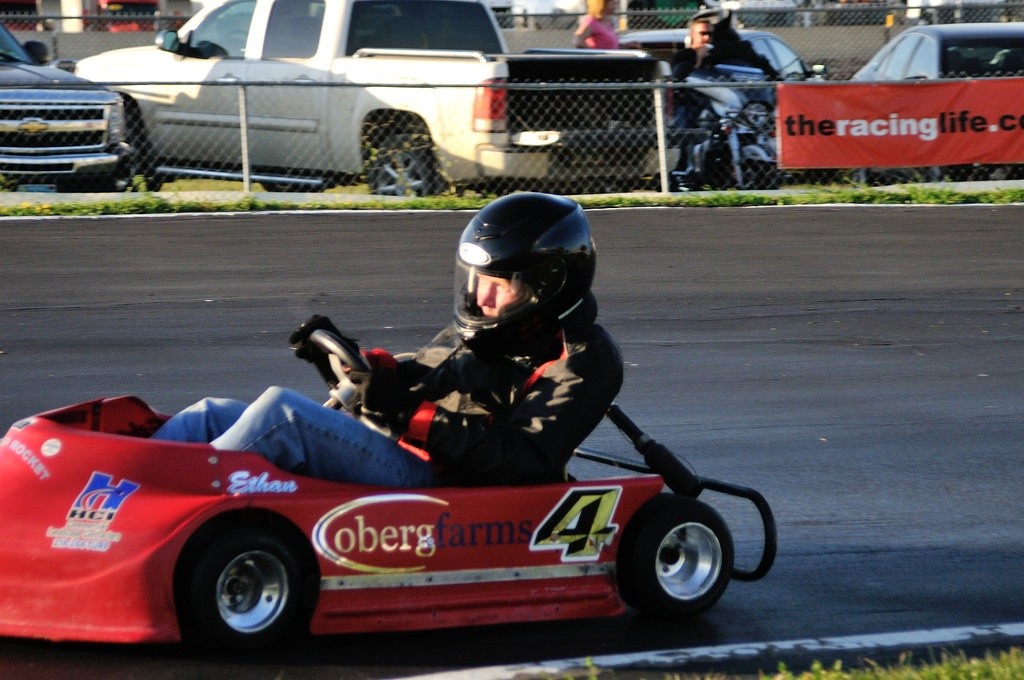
[454,192,596,359]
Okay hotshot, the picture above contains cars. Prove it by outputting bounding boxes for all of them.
[851,22,1024,79]
[620,30,827,82]
[1,22,161,192]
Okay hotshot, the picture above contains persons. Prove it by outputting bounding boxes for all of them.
[572,0,619,50]
[672,20,727,82]
[152,192,623,487]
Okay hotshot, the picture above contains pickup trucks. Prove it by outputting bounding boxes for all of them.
[75,0,682,198]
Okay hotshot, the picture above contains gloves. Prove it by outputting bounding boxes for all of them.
[349,361,419,436]
[288,314,359,382]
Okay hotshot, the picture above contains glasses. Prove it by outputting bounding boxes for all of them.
[699,30,713,36]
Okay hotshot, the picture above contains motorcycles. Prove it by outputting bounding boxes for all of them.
[645,47,805,190]
